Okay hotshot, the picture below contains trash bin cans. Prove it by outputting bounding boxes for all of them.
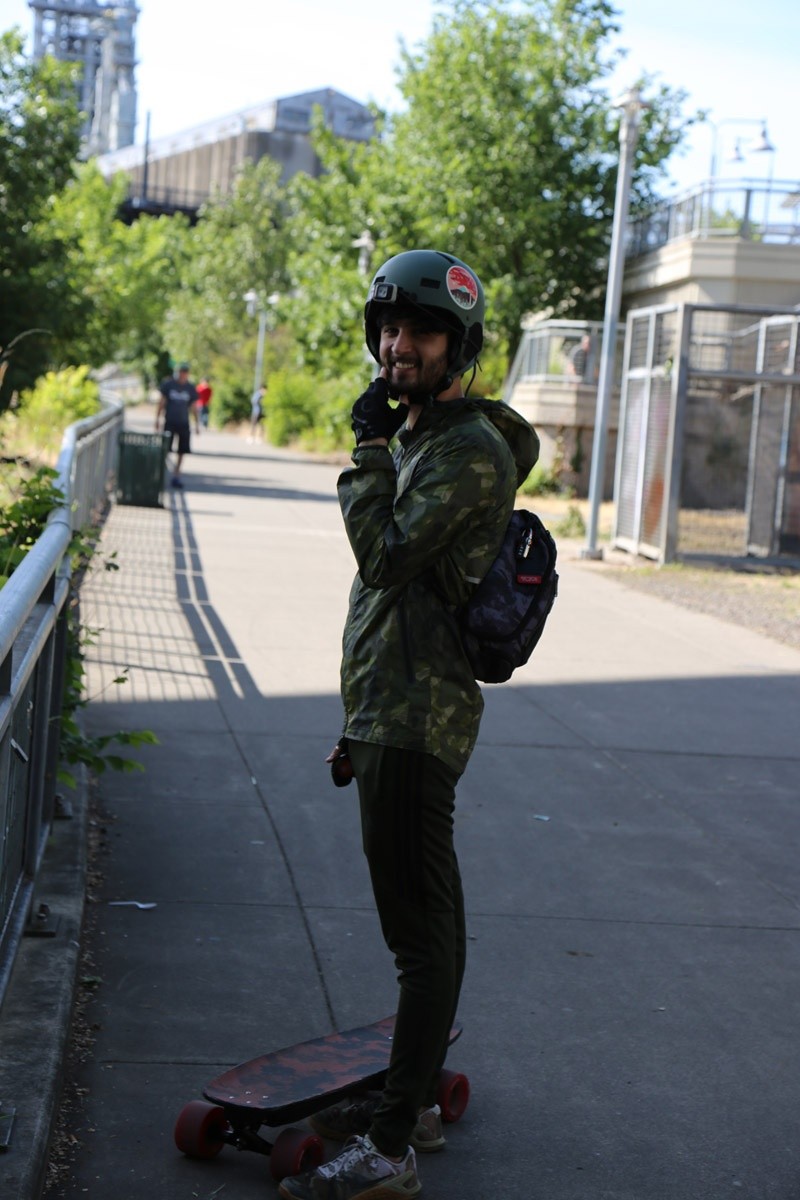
[116,430,172,508]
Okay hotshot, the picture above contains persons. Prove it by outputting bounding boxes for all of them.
[275,249,519,1200]
[250,386,269,447]
[155,361,201,489]
[155,351,175,430]
[195,376,212,433]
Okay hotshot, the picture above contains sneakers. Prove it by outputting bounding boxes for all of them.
[319,1096,447,1152]
[280,1134,422,1200]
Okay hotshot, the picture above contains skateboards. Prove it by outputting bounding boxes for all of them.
[171,1013,472,1176]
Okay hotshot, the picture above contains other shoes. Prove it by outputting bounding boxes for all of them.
[171,477,183,488]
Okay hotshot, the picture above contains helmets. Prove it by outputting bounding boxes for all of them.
[364,250,484,382]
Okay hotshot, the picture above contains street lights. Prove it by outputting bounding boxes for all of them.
[577,50,651,561]
[243,289,279,425]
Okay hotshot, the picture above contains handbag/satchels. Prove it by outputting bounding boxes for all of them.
[455,509,559,682]
